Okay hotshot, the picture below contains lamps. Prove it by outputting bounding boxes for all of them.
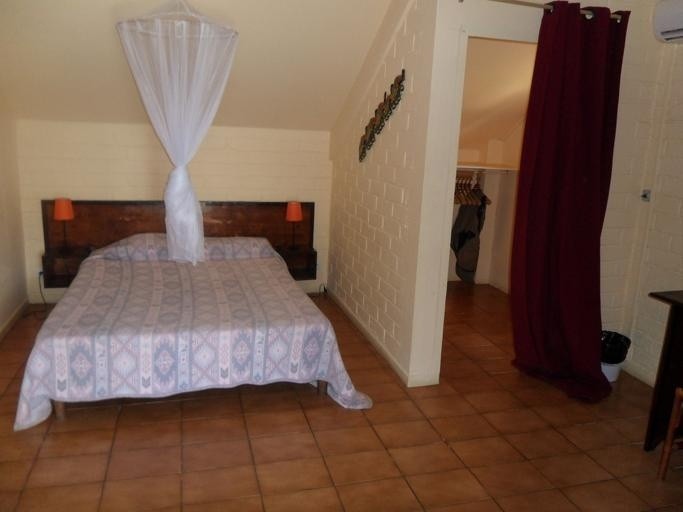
[52,197,76,255]
[286,200,304,251]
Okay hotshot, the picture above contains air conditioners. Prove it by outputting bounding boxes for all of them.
[652,0,683,45]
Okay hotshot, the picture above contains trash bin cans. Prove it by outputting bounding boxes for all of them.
[601,330,632,383]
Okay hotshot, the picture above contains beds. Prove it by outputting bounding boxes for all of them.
[10,231,374,433]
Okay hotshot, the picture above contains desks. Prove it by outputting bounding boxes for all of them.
[42,246,91,288]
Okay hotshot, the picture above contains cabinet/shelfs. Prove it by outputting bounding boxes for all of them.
[276,245,317,281]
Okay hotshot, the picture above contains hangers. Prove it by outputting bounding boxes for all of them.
[454,170,493,207]
